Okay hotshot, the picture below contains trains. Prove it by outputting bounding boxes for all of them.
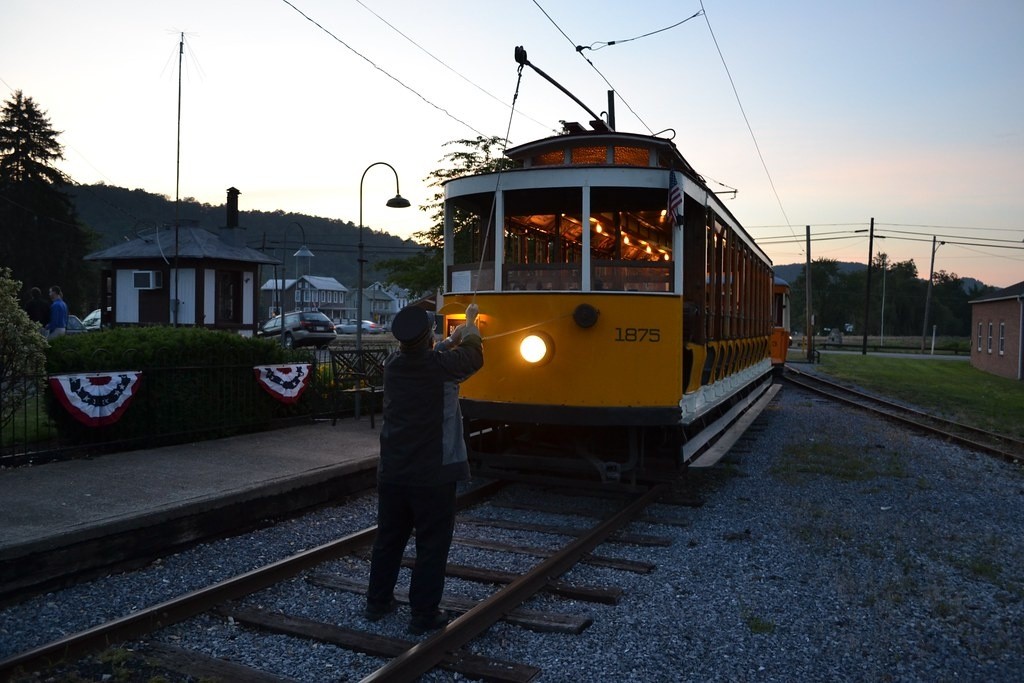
[437,44,790,474]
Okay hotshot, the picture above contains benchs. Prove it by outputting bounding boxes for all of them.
[330,347,388,428]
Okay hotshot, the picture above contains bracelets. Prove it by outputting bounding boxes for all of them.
[446,337,454,348]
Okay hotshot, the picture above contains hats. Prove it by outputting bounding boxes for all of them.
[391,306,435,347]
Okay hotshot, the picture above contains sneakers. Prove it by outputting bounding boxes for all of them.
[408,610,449,634]
[365,598,396,620]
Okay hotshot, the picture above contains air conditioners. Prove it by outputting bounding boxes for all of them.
[132,271,162,289]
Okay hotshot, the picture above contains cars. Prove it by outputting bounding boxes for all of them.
[257,311,337,349]
[334,319,382,334]
[44,307,113,334]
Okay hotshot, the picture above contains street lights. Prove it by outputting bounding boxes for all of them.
[920,236,946,354]
[355,162,411,419]
[281,222,316,348]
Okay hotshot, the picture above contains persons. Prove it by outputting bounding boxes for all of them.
[366,303,484,632]
[46,286,69,337]
[25,287,47,329]
